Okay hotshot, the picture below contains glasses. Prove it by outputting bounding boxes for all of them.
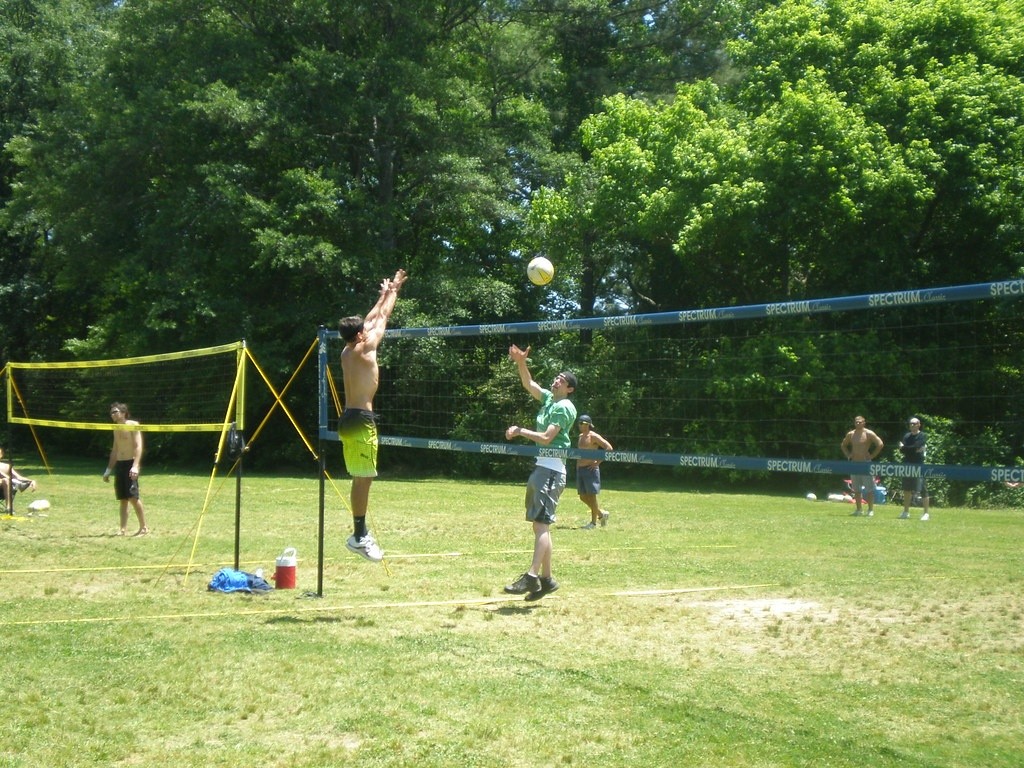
[110,410,120,414]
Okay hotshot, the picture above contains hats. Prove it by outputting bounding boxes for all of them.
[560,371,577,394]
[578,415,594,429]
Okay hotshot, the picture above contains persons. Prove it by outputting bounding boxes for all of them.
[0,448,36,500]
[504,344,578,602]
[102,402,151,538]
[575,415,614,530]
[337,268,408,562]
[841,416,883,517]
[898,417,929,521]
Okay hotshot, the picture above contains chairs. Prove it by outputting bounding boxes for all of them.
[0,488,18,515]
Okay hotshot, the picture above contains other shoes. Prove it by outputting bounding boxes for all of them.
[132,525,148,538]
[5,509,15,515]
[18,482,31,493]
[117,527,126,536]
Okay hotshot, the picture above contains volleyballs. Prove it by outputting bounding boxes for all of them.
[807,493,817,501]
[527,257,554,285]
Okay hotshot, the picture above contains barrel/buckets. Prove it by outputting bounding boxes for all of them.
[275,547,297,589]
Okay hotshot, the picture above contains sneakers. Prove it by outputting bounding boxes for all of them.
[526,577,559,601]
[920,512,930,521]
[346,531,383,562]
[504,573,541,595]
[598,511,609,525]
[866,509,874,517]
[581,522,596,530]
[898,511,910,519]
[851,510,864,516]
